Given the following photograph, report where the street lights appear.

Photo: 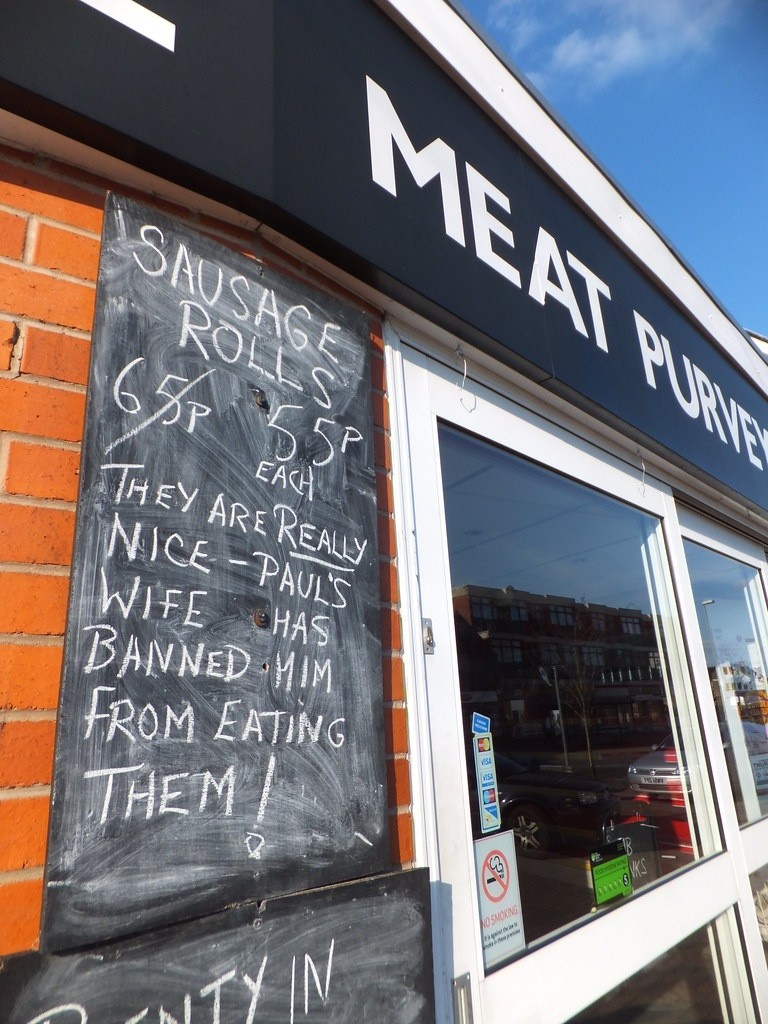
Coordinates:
[702,599,720,666]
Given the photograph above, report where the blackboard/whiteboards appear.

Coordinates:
[34,190,403,953]
[2,865,437,1024]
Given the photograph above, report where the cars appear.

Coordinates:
[465,744,621,860]
[625,720,768,800]
